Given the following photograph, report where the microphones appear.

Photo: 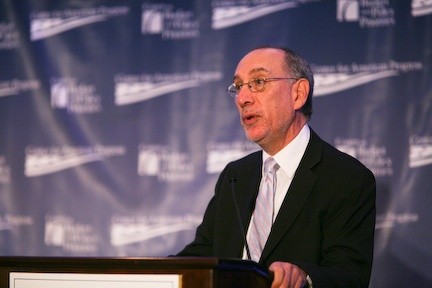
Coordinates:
[231,177,252,260]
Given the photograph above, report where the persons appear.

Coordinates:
[169,48,377,288]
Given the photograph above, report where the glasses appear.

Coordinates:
[225,77,298,98]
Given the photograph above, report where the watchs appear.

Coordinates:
[303,273,312,288]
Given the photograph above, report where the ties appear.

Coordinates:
[242,156,282,263]
